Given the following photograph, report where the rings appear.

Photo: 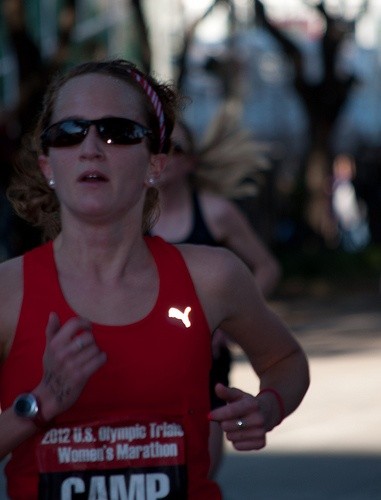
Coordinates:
[233,417,248,431]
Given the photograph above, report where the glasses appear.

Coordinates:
[38,118,155,148]
[167,142,188,156]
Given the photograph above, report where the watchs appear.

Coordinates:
[13,391,48,430]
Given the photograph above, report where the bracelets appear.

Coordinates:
[253,387,285,424]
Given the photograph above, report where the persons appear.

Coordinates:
[2,59,311,498]
[305,148,371,286]
[141,117,282,476]
